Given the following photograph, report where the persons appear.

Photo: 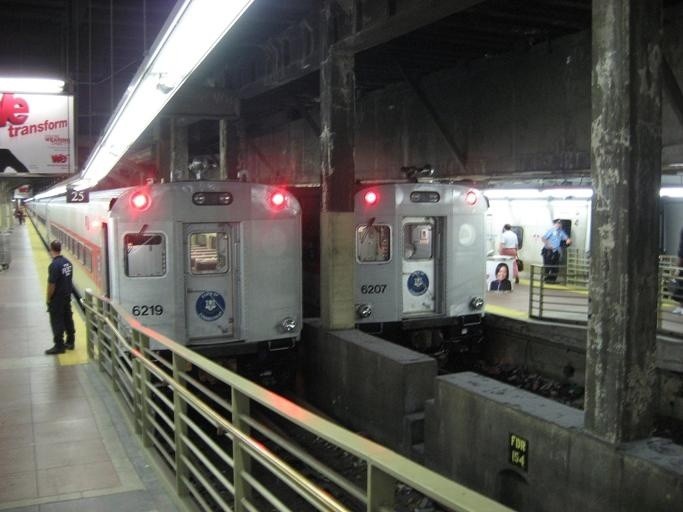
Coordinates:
[672,228,683,316]
[540,218,572,283]
[490,263,512,291]
[14,206,23,225]
[501,224,520,284]
[45,239,73,354]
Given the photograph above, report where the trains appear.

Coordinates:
[23,177,304,389]
[279,165,491,371]
[485,190,683,296]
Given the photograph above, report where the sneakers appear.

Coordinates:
[45,341,74,354]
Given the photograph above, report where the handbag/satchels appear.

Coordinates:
[515,259,523,271]
